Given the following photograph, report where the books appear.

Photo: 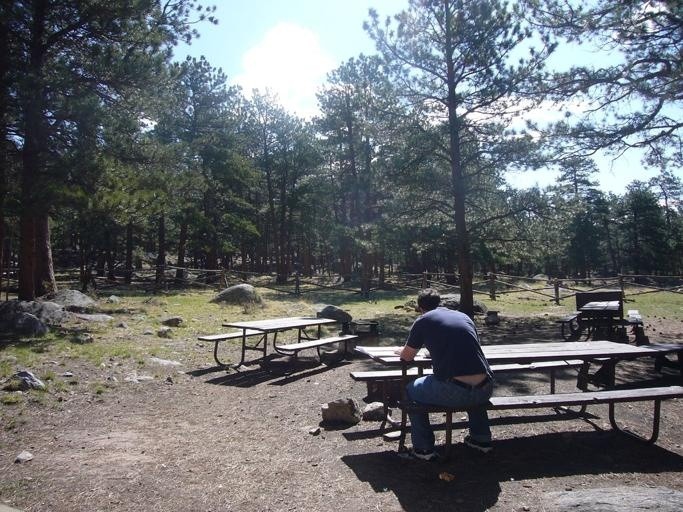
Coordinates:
[393,348,424,356]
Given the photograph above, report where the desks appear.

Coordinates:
[343,358,613,403]
[581,301,623,337]
[224,316,338,370]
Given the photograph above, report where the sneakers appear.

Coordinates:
[415,447,436,460]
[464,434,494,453]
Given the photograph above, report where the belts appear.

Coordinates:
[449,377,488,390]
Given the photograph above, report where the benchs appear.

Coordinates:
[396,383,681,451]
[272,332,362,373]
[198,329,270,367]
[556,309,582,335]
[625,307,644,338]
[351,338,654,434]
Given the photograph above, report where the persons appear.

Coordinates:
[399,287,495,463]
